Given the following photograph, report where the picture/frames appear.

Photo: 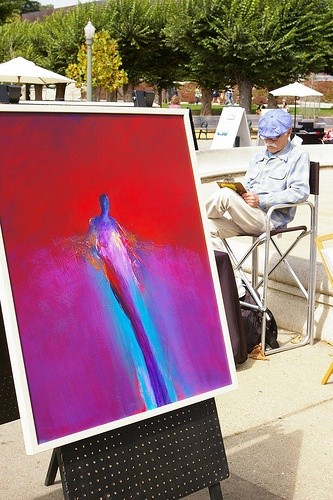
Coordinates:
[0,104,239,455]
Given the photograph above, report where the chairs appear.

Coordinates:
[219,161,320,356]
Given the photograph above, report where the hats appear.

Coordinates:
[258,109,293,138]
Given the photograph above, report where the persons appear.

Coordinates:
[282,99,288,109]
[257,101,265,110]
[171,92,180,105]
[194,86,200,104]
[323,129,333,140]
[212,87,234,105]
[205,108,310,301]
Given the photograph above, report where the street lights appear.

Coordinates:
[84,21,96,102]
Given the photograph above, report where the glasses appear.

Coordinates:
[259,134,281,141]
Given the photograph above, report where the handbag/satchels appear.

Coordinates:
[239,280,279,354]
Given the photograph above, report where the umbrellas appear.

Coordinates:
[269,82,323,128]
[0,56,77,87]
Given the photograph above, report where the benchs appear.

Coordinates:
[192,115,252,139]
